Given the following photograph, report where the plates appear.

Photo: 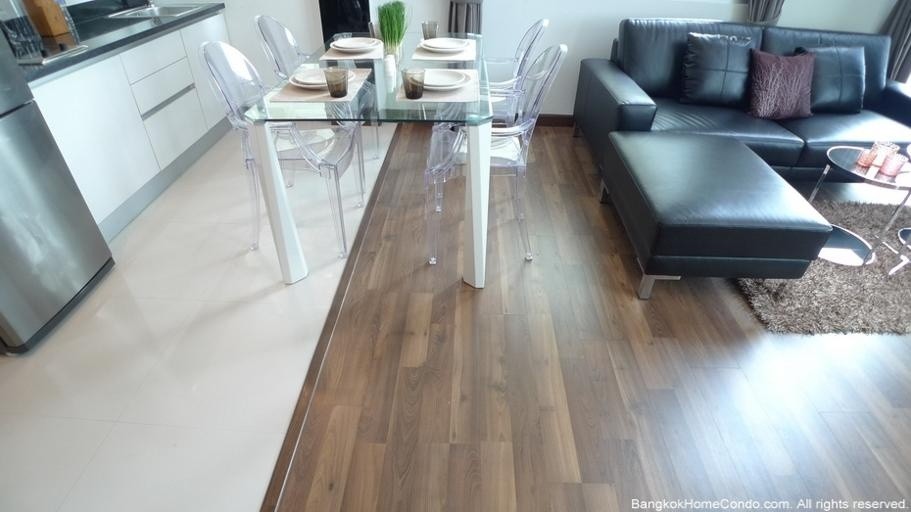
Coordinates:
[290,69,357,91]
[330,37,381,54]
[421,37,473,53]
[412,69,472,91]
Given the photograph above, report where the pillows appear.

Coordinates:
[748,44,816,123]
[799,45,868,116]
[675,27,757,106]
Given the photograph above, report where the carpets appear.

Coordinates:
[730,198,911,334]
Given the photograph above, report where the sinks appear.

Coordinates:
[104,4,206,18]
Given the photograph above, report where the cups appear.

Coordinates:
[422,20,439,40]
[402,66,426,99]
[325,66,348,98]
[367,20,386,41]
[859,141,909,176]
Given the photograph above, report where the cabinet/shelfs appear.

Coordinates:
[120,31,216,187]
[183,8,244,142]
[25,46,160,232]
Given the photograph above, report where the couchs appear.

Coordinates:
[570,15,911,301]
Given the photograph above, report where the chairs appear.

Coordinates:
[425,43,569,265]
[430,18,554,186]
[197,40,363,261]
[256,12,381,195]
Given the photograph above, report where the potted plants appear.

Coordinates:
[376,2,410,50]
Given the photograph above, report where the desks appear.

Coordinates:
[245,30,495,287]
[804,145,910,269]
